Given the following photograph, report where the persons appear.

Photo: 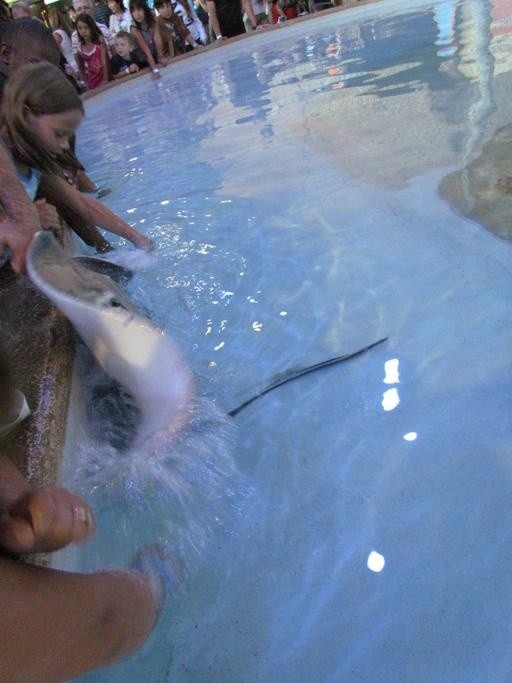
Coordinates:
[0,0,343,91]
[0,61,156,681]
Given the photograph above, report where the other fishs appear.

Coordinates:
[22,229,390,488]
